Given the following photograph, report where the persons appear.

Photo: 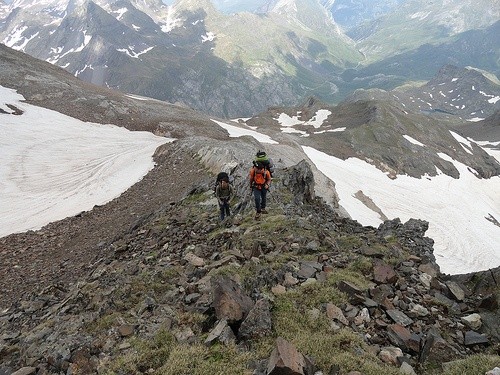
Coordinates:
[249,163,271,219]
[216,178,234,220]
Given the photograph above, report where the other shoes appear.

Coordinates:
[255,213,261,218]
[261,210,268,214]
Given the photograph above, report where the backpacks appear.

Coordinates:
[253,149,274,186]
[214,172,231,201]
